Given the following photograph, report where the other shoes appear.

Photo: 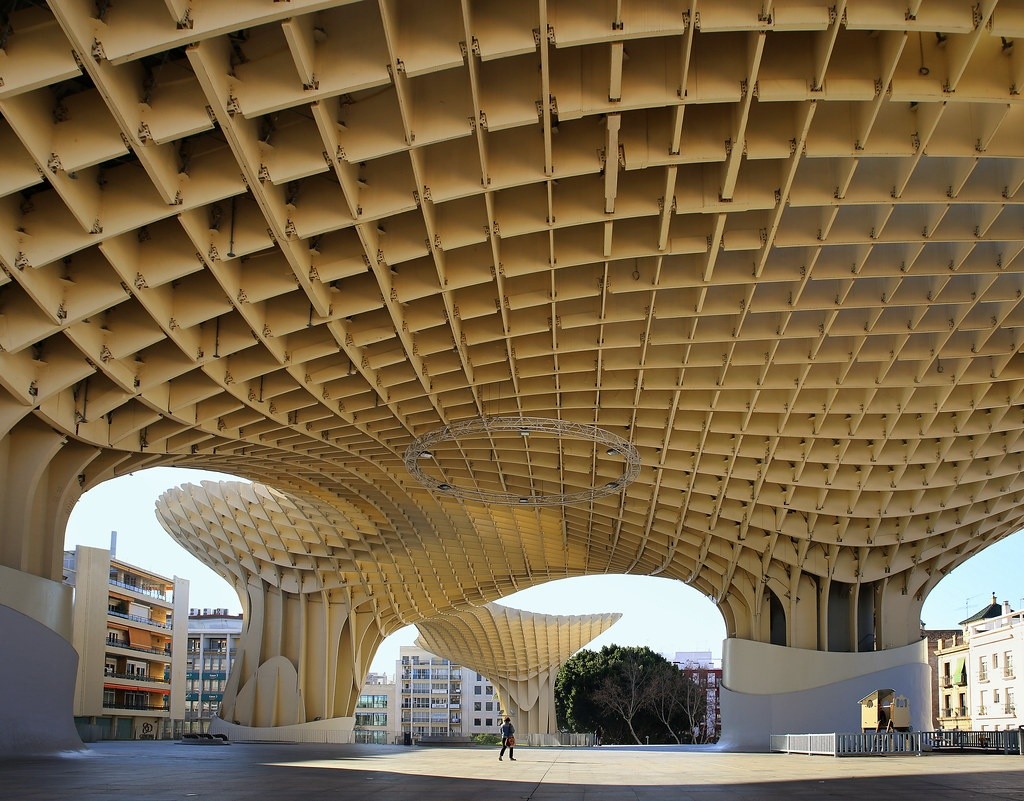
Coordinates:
[499,756,503,761]
[510,758,516,761]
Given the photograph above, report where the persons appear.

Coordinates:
[499,716,516,761]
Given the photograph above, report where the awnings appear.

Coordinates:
[953,658,964,682]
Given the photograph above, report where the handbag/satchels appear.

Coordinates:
[505,737,515,748]
